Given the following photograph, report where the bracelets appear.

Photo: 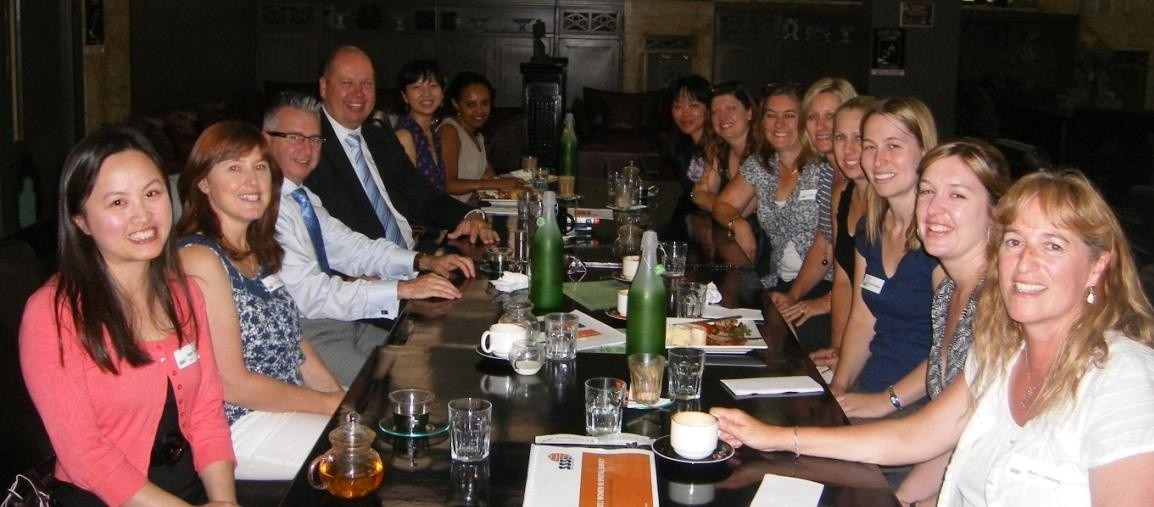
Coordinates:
[727,215,741,238]
[794,425,800,458]
[886,386,904,413]
[414,250,426,273]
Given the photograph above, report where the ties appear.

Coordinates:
[345,133,409,250]
[293,185,334,277]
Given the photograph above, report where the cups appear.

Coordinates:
[546,360,576,391]
[481,324,528,357]
[608,171,618,195]
[451,463,490,506]
[661,240,688,276]
[509,343,544,375]
[449,397,492,462]
[389,437,435,473]
[667,348,705,399]
[628,353,665,404]
[677,282,707,318]
[617,289,632,316]
[670,411,719,460]
[623,256,640,278]
[520,156,536,171]
[668,483,715,505]
[533,166,548,191]
[531,202,543,217]
[517,193,531,220]
[585,377,627,435]
[488,248,509,280]
[480,375,510,395]
[388,389,435,429]
[616,182,631,207]
[544,313,578,359]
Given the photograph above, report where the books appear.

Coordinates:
[563,209,614,224]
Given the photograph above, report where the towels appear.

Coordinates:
[488,269,531,294]
[566,208,614,220]
[702,280,724,303]
[720,375,826,396]
[510,169,532,181]
[749,471,825,507]
[701,303,764,322]
[479,206,519,215]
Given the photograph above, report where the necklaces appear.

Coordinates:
[239,250,261,274]
[1019,344,1050,406]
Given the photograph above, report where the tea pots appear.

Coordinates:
[620,160,644,195]
[308,412,384,497]
[616,217,644,259]
[498,301,540,346]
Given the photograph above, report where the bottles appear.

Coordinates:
[529,190,565,309]
[626,230,666,365]
[559,112,578,179]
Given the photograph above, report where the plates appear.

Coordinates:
[481,191,517,207]
[605,308,631,320]
[379,418,449,437]
[652,463,735,484]
[612,274,636,283]
[651,435,734,464]
[475,345,512,360]
[664,317,769,354]
[500,173,557,185]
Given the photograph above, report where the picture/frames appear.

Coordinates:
[870,26,906,76]
[899,0,935,28]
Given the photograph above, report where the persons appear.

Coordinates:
[303,44,502,252]
[709,74,832,304]
[805,97,883,368]
[833,139,1014,507]
[392,60,446,194]
[824,97,950,398]
[692,81,769,258]
[171,120,350,482]
[667,74,714,195]
[765,77,858,349]
[18,125,233,506]
[435,76,525,202]
[705,172,1154,507]
[262,89,475,388]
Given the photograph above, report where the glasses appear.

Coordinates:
[266,130,327,144]
